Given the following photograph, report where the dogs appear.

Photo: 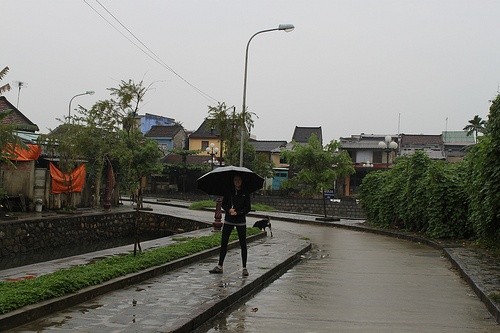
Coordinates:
[253,215,272,238]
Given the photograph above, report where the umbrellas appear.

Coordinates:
[196,165,264,193]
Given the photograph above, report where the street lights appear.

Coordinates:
[206,143,220,170]
[378,135,398,169]
[239,23,294,166]
[69,91,95,123]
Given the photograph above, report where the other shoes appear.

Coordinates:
[242,268,249,276]
[208,266,224,274]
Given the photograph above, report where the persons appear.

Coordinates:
[208,173,251,277]
